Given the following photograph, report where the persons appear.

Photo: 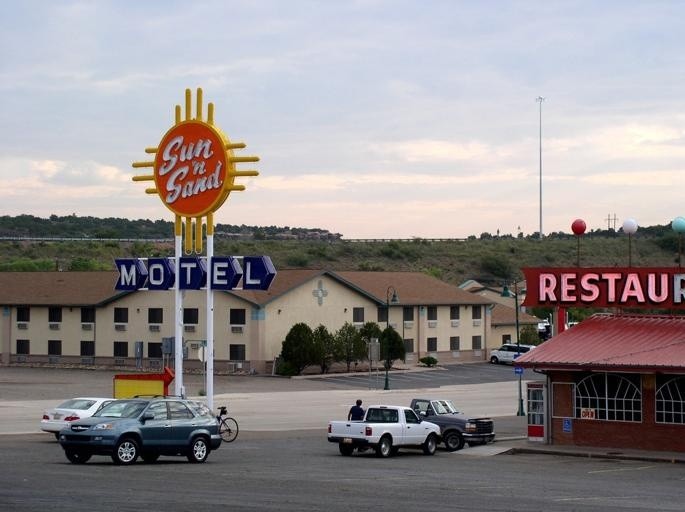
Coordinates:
[348,399,365,421]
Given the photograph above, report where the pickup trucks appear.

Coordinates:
[409,397,498,453]
[325,404,444,457]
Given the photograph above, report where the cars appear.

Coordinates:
[41,396,126,442]
[538,316,581,340]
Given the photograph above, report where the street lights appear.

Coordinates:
[384,284,401,392]
[499,275,530,416]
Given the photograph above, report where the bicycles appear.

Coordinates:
[213,406,238,443]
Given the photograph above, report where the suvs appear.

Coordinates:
[57,393,223,466]
[491,343,537,366]
